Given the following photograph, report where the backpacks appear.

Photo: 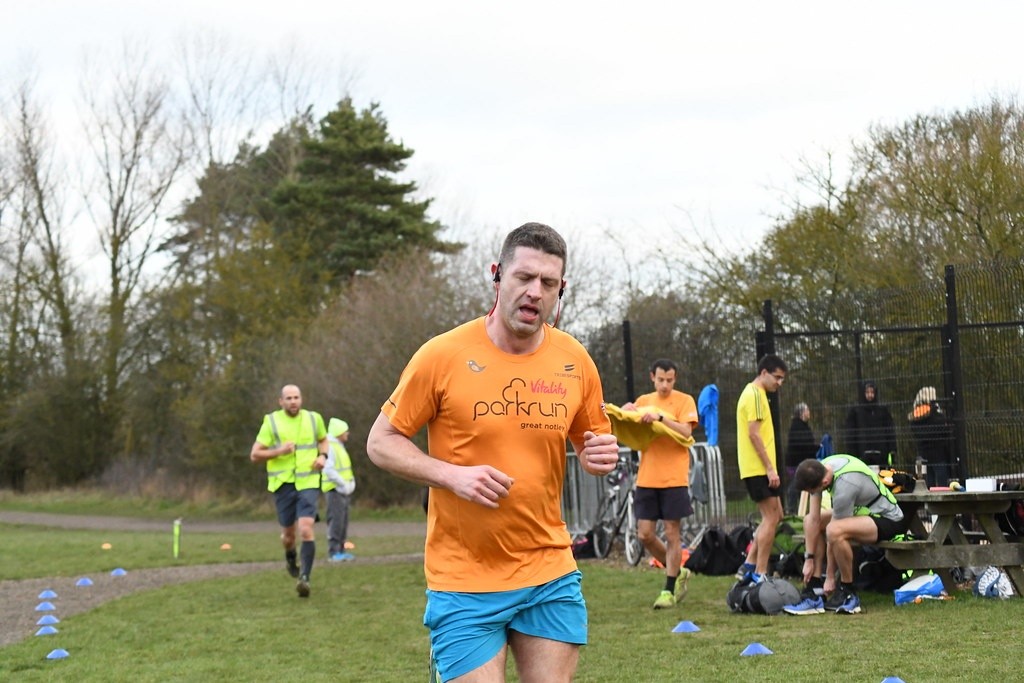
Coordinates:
[686,527,752,575]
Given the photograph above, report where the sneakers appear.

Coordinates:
[734,563,753,584]
[673,567,690,602]
[653,590,677,608]
[836,593,862,613]
[782,596,826,615]
[749,574,771,587]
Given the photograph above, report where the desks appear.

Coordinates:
[894,490,1024,598]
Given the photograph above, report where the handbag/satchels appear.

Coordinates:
[726,577,802,614]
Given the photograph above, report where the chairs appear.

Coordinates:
[733,484,804,579]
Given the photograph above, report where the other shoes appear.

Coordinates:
[328,551,354,563]
[296,581,309,597]
[287,563,299,577]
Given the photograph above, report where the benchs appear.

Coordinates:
[791,530,1024,551]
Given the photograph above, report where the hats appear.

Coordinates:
[328,417,348,438]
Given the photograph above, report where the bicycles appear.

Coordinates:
[592,457,643,566]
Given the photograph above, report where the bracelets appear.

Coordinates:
[804,552,814,559]
[318,453,328,459]
[658,412,663,421]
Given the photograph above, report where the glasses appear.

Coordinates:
[770,371,784,382]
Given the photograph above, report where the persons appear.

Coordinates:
[322,417,355,561]
[845,381,897,465]
[251,384,329,597]
[912,387,949,491]
[619,359,698,607]
[787,404,820,515]
[783,454,907,614]
[369,222,619,683]
[735,355,785,581]
